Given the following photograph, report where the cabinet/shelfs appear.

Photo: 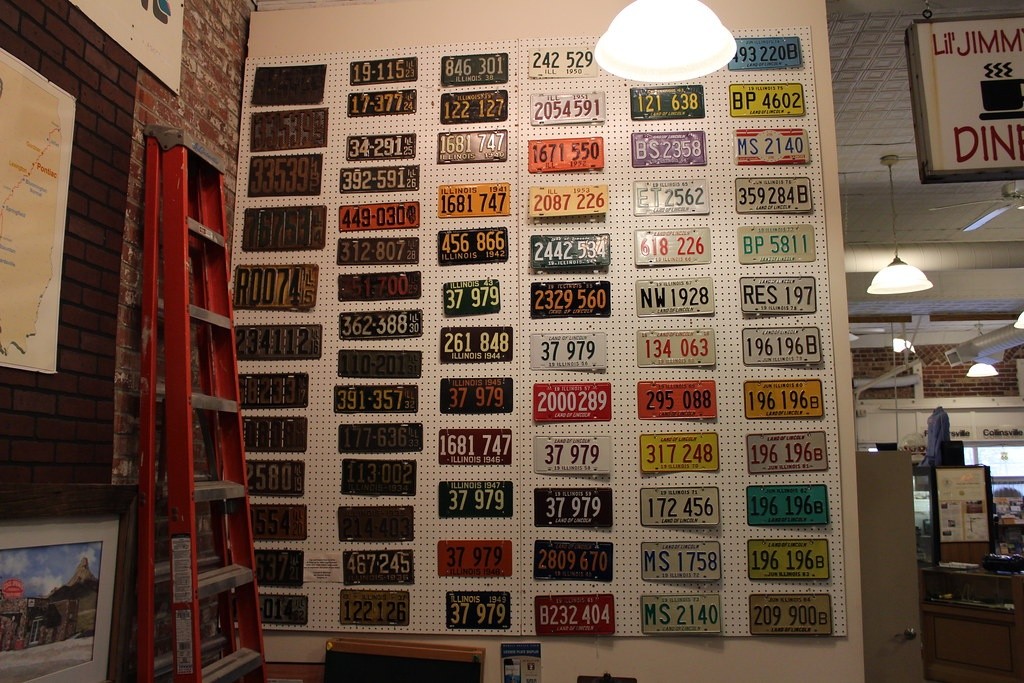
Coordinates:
[918,563,1024,683]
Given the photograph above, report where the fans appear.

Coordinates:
[928,182,1024,232]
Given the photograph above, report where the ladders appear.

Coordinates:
[135,124,269,683]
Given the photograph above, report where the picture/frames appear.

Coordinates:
[0,481,164,683]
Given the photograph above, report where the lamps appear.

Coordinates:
[594,0,738,83]
[965,320,999,377]
[866,155,935,295]
